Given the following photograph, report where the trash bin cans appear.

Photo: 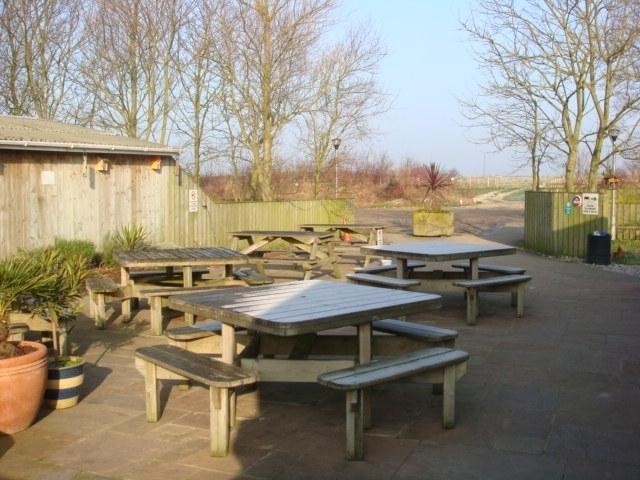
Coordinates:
[587,233,611,265]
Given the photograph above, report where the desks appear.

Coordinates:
[298,224,386,246]
[168,278,444,458]
[113,246,249,325]
[361,239,516,324]
[230,230,341,280]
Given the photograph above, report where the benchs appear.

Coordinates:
[130,269,210,283]
[451,262,526,307]
[134,345,256,455]
[375,319,457,350]
[345,272,421,290]
[164,322,246,350]
[316,346,468,460]
[139,283,241,335]
[85,276,118,331]
[354,263,426,279]
[453,273,532,326]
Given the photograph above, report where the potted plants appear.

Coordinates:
[412,162,455,236]
[17,238,95,410]
[0,255,65,435]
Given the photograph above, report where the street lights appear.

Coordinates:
[332,139,341,197]
[608,129,620,240]
